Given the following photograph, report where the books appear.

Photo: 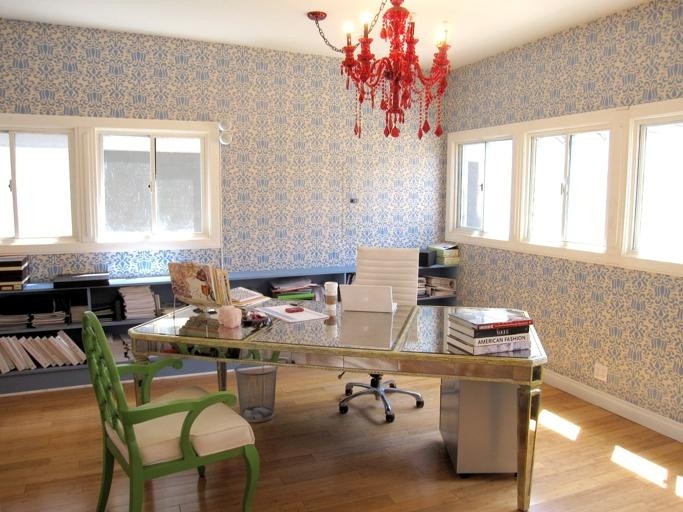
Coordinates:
[92,305,113,322]
[269,277,324,301]
[70,305,89,322]
[447,307,531,357]
[418,244,460,300]
[31,311,70,329]
[168,262,272,307]
[119,285,188,320]
[2,314,30,331]
[105,333,137,363]
[2,330,87,374]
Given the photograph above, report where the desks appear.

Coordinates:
[128,299,548,509]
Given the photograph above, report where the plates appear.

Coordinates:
[241,313,268,326]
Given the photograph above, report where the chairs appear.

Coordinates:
[338,246,424,422]
[81,311,260,512]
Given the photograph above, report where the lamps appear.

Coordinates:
[341,0,453,138]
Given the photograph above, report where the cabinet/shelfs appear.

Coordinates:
[0,264,461,396]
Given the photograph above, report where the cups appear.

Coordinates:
[323,281,338,310]
[217,305,241,329]
[322,311,337,339]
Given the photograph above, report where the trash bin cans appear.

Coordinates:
[235,364,278,423]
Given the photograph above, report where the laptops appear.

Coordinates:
[340,305,398,351]
[339,283,398,312]
